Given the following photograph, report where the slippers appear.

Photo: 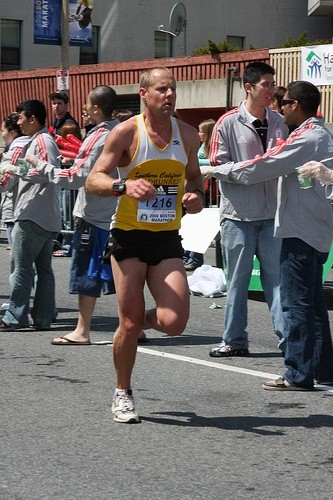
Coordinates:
[51,332,91,345]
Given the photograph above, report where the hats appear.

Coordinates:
[48,91,69,102]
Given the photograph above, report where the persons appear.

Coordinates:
[25,85,149,345]
[84,67,204,423]
[268,86,299,135]
[0,99,61,332]
[199,81,333,391]
[209,62,289,358]
[295,160,333,185]
[48,91,135,168]
[52,123,82,257]
[181,119,215,271]
[0,113,59,323]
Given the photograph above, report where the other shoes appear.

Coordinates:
[262,376,310,391]
[53,250,70,256]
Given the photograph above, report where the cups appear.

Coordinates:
[295,166,314,189]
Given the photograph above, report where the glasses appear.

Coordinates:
[252,81,276,90]
[280,99,294,106]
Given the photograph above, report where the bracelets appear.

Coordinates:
[193,189,204,199]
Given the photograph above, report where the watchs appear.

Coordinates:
[112,178,129,194]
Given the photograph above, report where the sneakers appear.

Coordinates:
[182,255,204,270]
[210,341,249,356]
[111,388,141,423]
[0,321,17,331]
